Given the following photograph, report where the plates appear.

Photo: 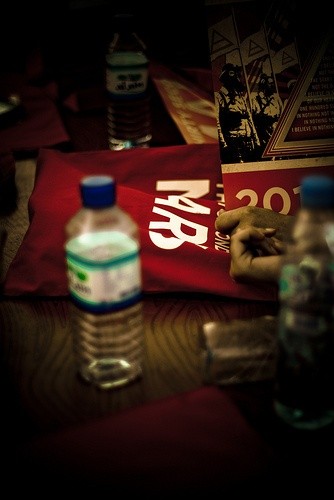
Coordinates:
[0,97,22,122]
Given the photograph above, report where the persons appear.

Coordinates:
[214,62,298,164]
[215,205,297,287]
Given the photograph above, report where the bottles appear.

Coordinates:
[105,12,153,151]
[64,175,145,390]
[273,176,334,430]
